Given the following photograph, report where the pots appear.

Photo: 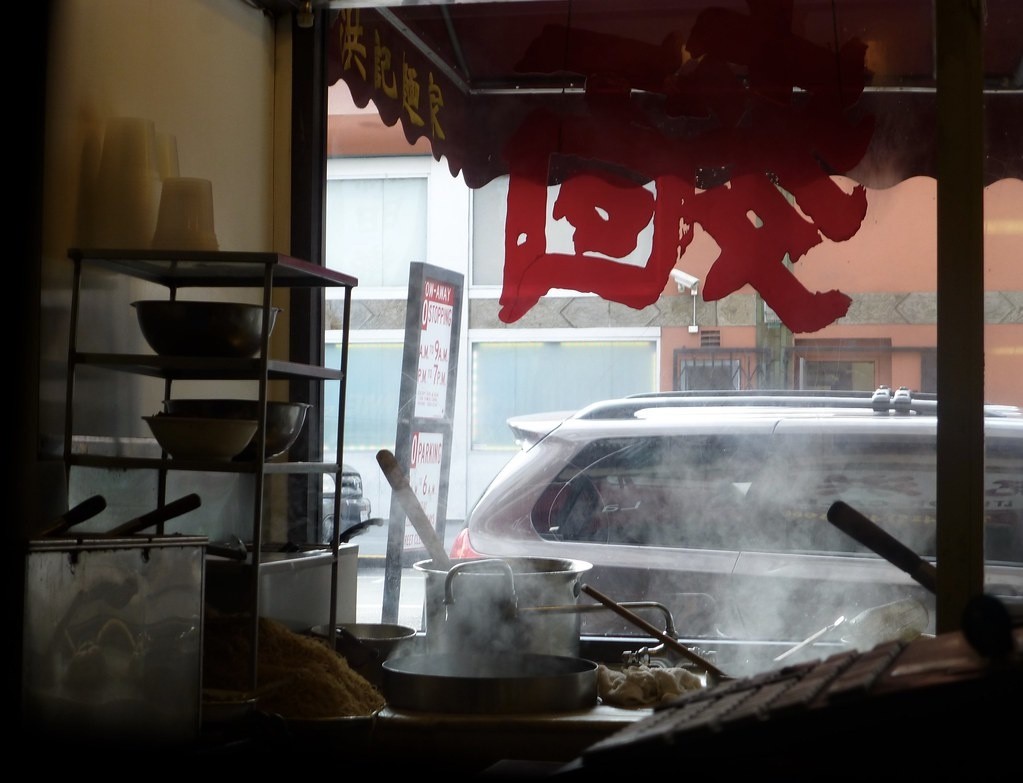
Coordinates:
[312,621,416,682]
[380,653,598,716]
[417,555,594,654]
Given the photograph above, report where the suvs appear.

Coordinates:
[446,390,1023,671]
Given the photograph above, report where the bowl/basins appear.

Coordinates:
[161,399,314,461]
[145,412,259,465]
[129,299,283,359]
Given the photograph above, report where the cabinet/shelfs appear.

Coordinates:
[59,252,358,738]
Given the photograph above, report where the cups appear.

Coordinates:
[88,114,219,252]
